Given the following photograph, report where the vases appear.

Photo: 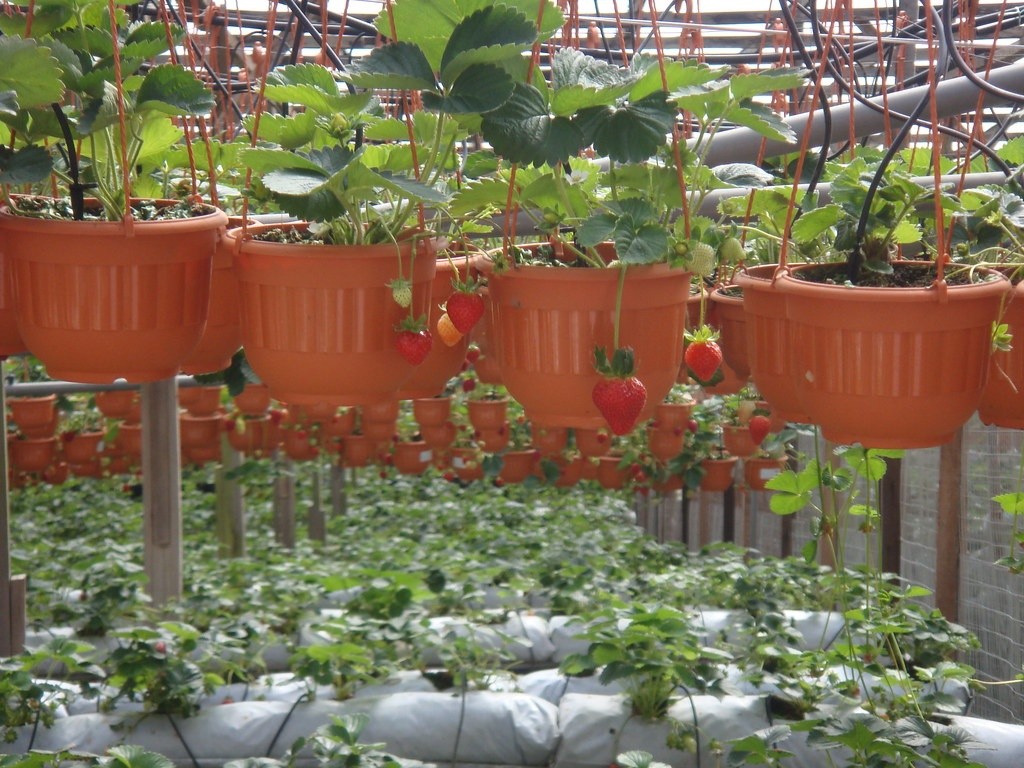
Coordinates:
[179,386,225,416]
[285,402,338,420]
[5,418,791,492]
[363,400,400,424]
[178,217,261,375]
[234,387,271,416]
[95,390,136,418]
[732,261,809,401]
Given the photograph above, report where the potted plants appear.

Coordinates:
[655,383,696,434]
[718,389,770,458]
[708,184,836,385]
[220,0,568,384]
[0,355,58,437]
[0,0,229,386]
[775,155,1013,447]
[462,384,509,431]
[412,377,455,427]
[482,49,789,431]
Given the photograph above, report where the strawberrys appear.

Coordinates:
[219,277,771,497]
